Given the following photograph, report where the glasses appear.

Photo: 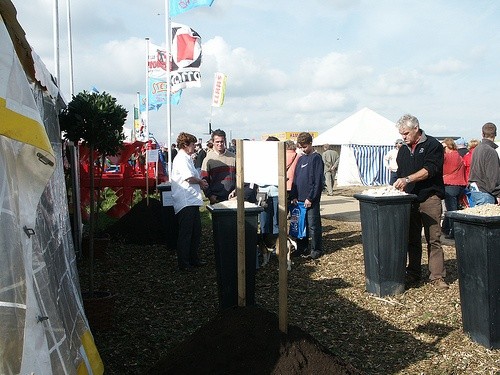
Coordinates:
[443,146,447,150]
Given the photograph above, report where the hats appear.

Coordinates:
[396,138,404,143]
[456,138,467,146]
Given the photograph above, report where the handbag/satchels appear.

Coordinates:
[288,201,308,238]
[256,192,269,208]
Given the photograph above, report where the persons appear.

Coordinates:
[201,129,237,204]
[384,138,404,185]
[281,140,299,217]
[159,139,236,173]
[468,123,500,207]
[168,133,208,275]
[258,137,285,257]
[439,135,481,240]
[391,115,449,291]
[289,133,323,262]
[242,138,258,204]
[322,144,339,196]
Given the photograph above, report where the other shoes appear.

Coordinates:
[405,272,424,283]
[293,243,308,256]
[327,194,334,196]
[430,277,449,289]
[180,264,192,272]
[445,233,454,239]
[300,248,324,259]
[191,260,207,266]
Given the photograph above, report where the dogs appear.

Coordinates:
[256,233,297,272]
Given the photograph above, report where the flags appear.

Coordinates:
[170,21,203,95]
[148,77,182,105]
[138,95,161,113]
[148,43,171,81]
[130,108,146,144]
[209,72,225,107]
[168,0,214,17]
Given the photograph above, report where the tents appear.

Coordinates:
[316,107,408,188]
